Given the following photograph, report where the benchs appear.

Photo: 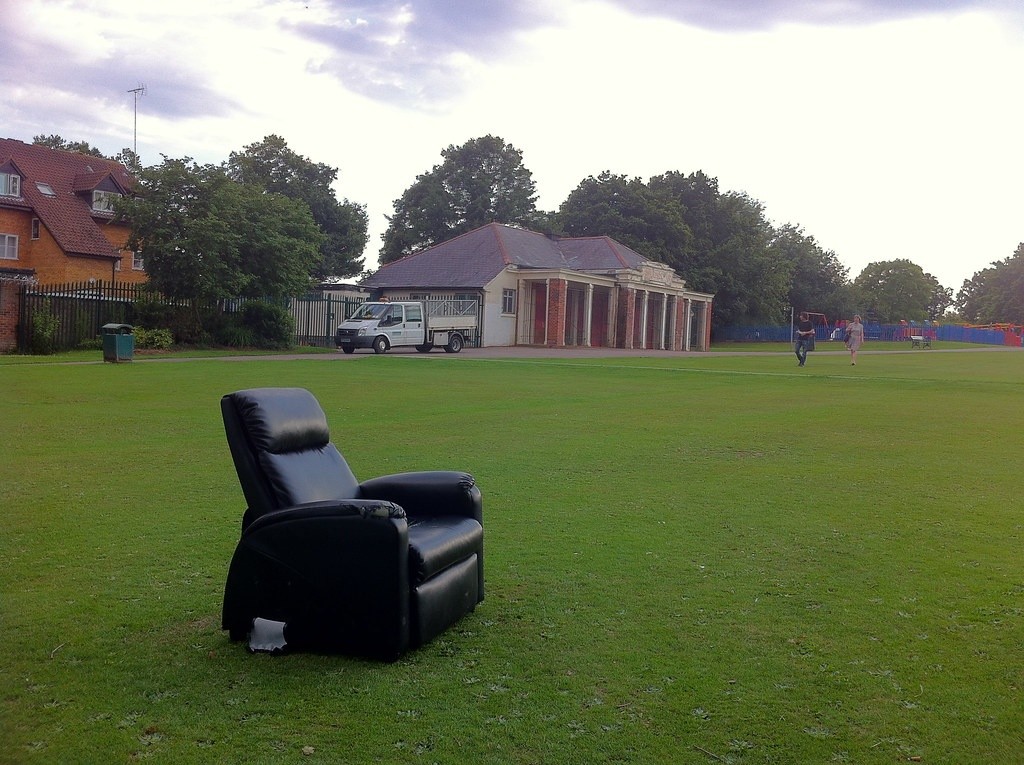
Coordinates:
[911,336,932,349]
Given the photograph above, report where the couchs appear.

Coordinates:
[217,388,485,665]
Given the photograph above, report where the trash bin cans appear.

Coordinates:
[102,323,135,363]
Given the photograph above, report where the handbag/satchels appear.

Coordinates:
[843,333,850,343]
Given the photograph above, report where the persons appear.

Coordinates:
[846,315,864,366]
[794,312,815,367]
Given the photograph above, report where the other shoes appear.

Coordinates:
[851,362,855,365]
[798,362,802,365]
[801,363,805,367]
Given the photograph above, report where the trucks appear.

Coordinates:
[330,295,477,354]
